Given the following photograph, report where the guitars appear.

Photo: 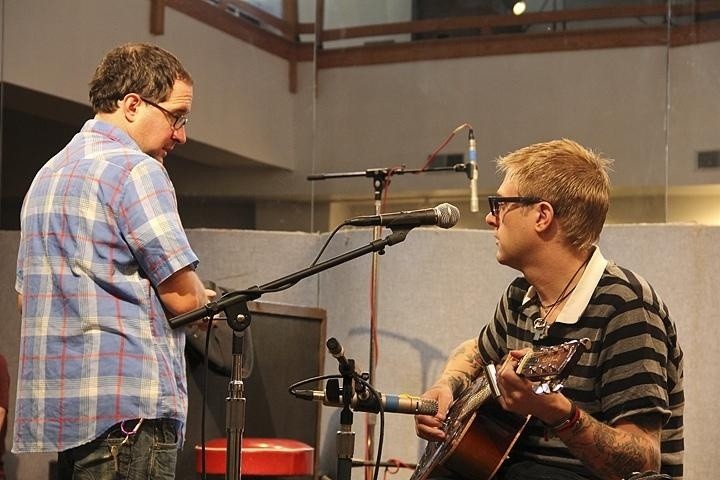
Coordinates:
[411,336,592,480]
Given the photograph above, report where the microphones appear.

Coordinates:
[294,387,439,416]
[344,201,461,230]
[467,128,479,213]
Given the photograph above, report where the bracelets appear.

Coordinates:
[546,398,576,431]
[552,406,581,434]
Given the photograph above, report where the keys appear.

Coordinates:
[110,444,122,473]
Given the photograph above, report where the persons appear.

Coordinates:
[409,134,685,479]
[7,42,215,480]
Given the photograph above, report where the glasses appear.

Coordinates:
[118,90,190,132]
[486,190,558,218]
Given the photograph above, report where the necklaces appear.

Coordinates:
[538,286,576,308]
[533,255,592,341]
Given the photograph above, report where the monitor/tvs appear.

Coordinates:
[178,305,329,477]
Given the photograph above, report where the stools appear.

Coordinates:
[193,435,316,480]
